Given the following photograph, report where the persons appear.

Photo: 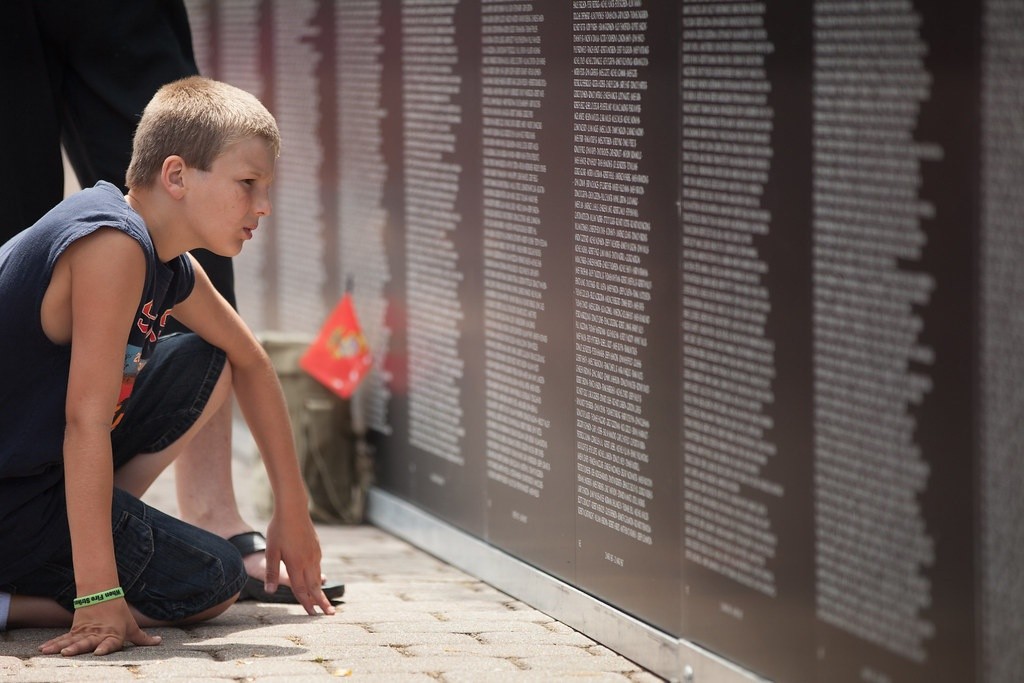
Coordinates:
[0,1,346,601]
[0,77,335,655]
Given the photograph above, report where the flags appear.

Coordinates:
[302,292,375,402]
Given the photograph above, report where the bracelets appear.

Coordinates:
[74,587,125,608]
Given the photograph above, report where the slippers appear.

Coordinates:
[229,532,345,604]
